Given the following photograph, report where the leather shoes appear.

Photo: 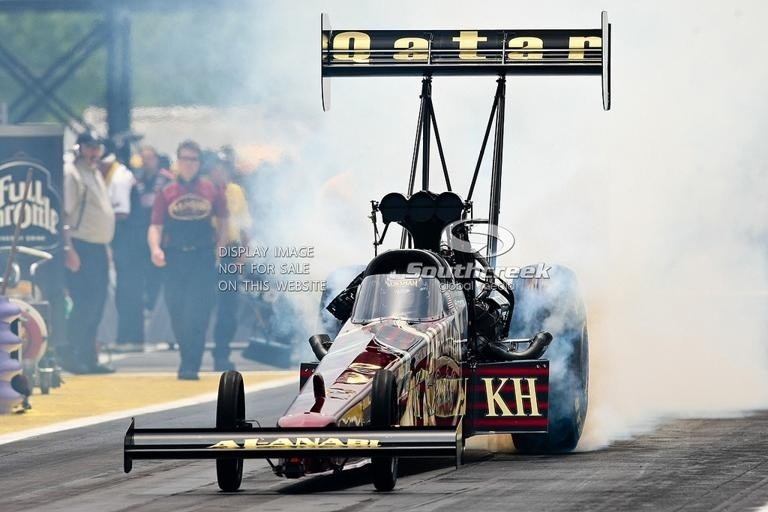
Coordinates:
[63,343,238,381]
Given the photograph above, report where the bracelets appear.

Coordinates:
[62,243,77,252]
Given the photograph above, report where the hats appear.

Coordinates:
[76,130,105,147]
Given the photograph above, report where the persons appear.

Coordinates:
[200,150,256,373]
[367,265,428,318]
[60,128,116,377]
[144,138,231,381]
[101,130,306,351]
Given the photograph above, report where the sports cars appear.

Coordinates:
[120,9,617,496]
